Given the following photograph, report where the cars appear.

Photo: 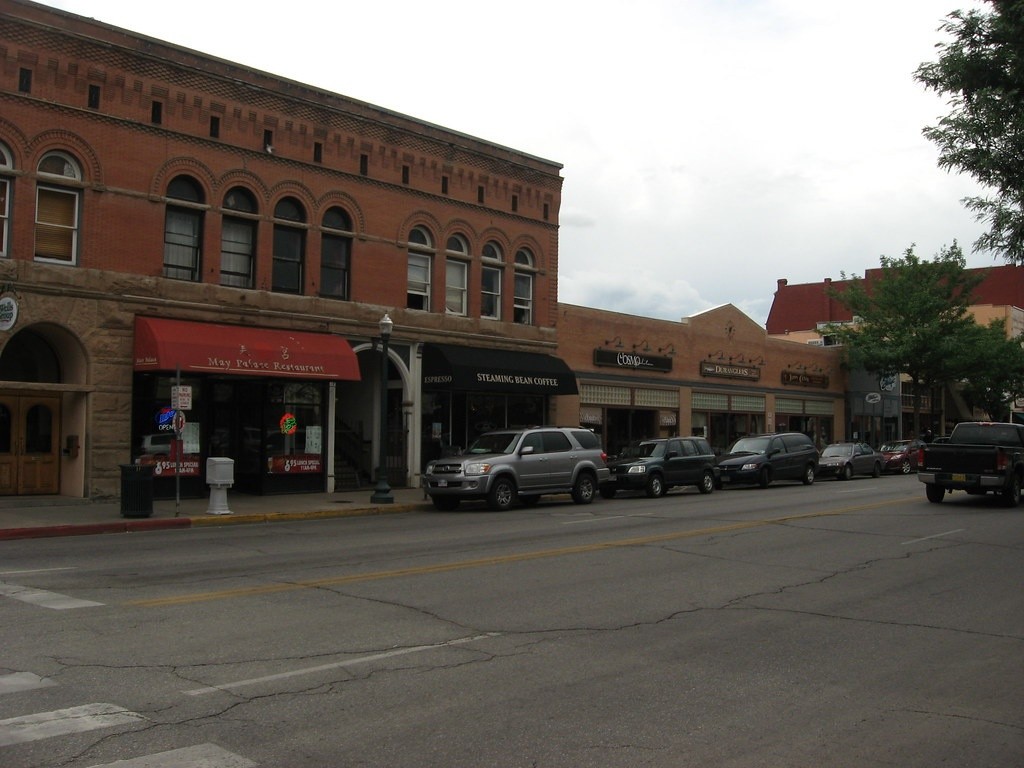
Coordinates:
[819,441,886,480]
[878,437,950,474]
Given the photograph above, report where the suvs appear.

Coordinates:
[422,425,609,511]
[600,436,721,499]
[714,432,820,490]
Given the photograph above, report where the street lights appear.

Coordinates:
[371,309,394,504]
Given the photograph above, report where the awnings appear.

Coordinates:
[133,316,361,380]
[421,342,578,395]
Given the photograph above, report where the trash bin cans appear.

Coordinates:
[119,462,158,518]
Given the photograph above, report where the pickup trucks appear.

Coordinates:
[918,421,1024,507]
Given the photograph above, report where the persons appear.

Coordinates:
[923,430,934,443]
